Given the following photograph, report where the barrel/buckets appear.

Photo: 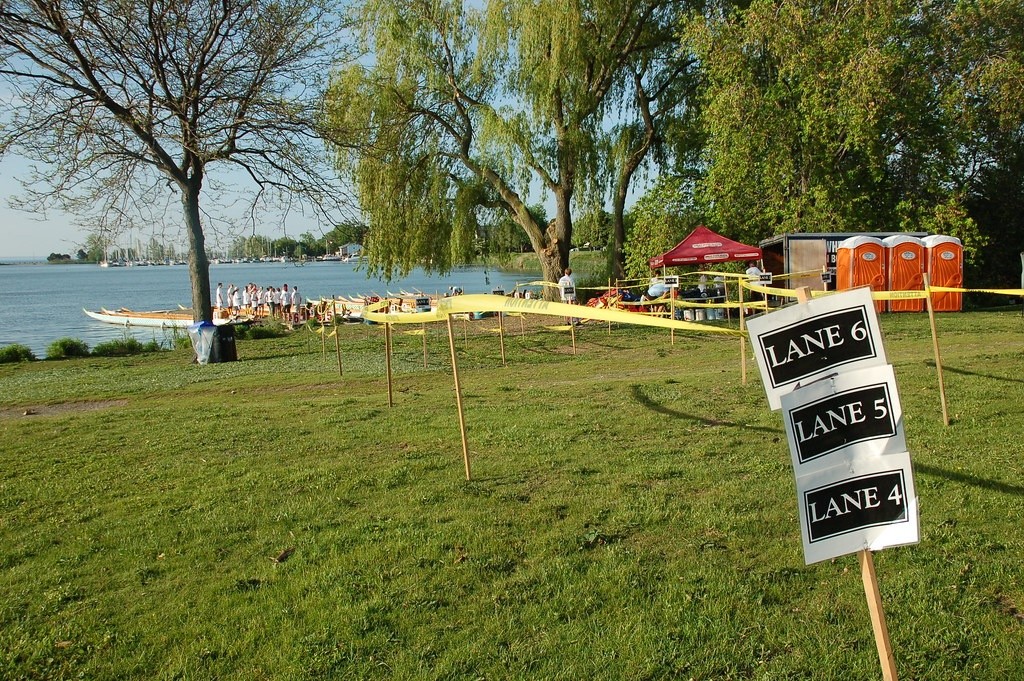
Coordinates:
[695,309,706,321]
[715,309,725,320]
[473,310,482,319]
[707,308,716,321]
[683,309,694,321]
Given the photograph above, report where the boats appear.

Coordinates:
[80,286,470,326]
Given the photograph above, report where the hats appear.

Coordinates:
[234,287,238,290]
[449,287,452,290]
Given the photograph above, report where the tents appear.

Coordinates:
[647,223,764,322]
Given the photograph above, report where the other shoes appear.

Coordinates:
[568,322,571,325]
[576,322,583,326]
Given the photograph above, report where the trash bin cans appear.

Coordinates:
[187,320,237,365]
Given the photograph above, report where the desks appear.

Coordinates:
[682,295,726,320]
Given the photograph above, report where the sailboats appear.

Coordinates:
[99,232,189,267]
[207,236,369,264]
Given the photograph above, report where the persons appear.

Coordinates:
[243,282,302,320]
[647,282,670,317]
[746,259,765,282]
[697,268,710,292]
[444,292,451,298]
[649,270,661,312]
[233,287,241,315]
[558,268,576,303]
[712,269,724,288]
[217,283,223,311]
[449,286,462,296]
[227,283,234,315]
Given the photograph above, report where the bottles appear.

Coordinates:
[717,288,720,296]
